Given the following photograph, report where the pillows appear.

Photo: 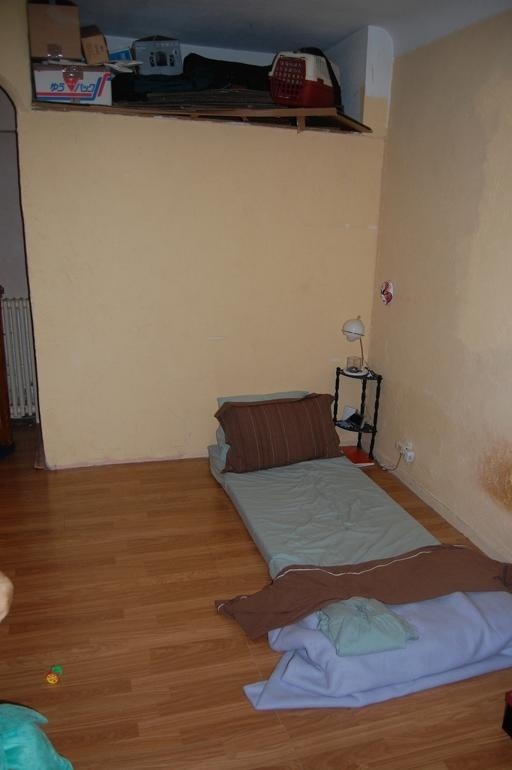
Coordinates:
[215,392,343,473]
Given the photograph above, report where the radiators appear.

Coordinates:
[0,297,39,420]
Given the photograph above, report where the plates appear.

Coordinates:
[343,366,369,377]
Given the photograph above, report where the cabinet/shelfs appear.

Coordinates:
[332,365,382,460]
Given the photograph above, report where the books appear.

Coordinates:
[340,444,377,467]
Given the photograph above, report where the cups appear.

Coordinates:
[347,355,363,371]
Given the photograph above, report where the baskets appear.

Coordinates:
[269,51,343,107]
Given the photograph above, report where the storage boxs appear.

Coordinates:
[28,4,184,107]
[268,43,341,107]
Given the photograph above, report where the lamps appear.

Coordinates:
[341,313,369,376]
